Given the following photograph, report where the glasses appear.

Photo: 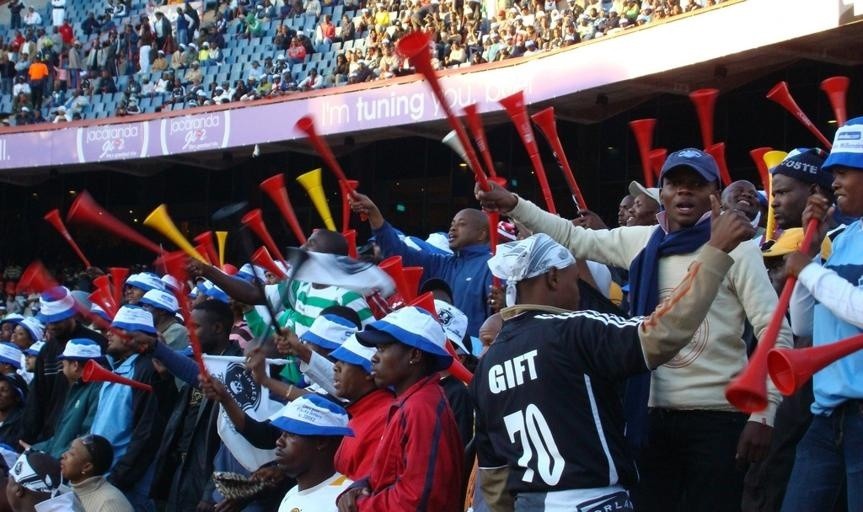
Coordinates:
[81,434,97,466]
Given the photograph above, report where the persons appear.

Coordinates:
[1,1,727,132]
[1,112,863,512]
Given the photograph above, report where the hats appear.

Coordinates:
[820,117,863,176]
[434,299,470,355]
[270,394,353,436]
[1,372,27,400]
[659,148,721,191]
[161,274,180,290]
[17,317,45,341]
[112,305,156,333]
[327,332,378,374]
[628,180,661,205]
[231,264,266,283]
[34,286,77,323]
[197,280,229,304]
[58,338,104,361]
[139,289,178,313]
[188,286,198,298]
[23,341,46,356]
[302,315,358,349]
[89,301,113,323]
[0,312,24,323]
[126,272,165,291]
[365,306,453,371]
[0,342,22,368]
[356,329,396,347]
[769,147,810,174]
